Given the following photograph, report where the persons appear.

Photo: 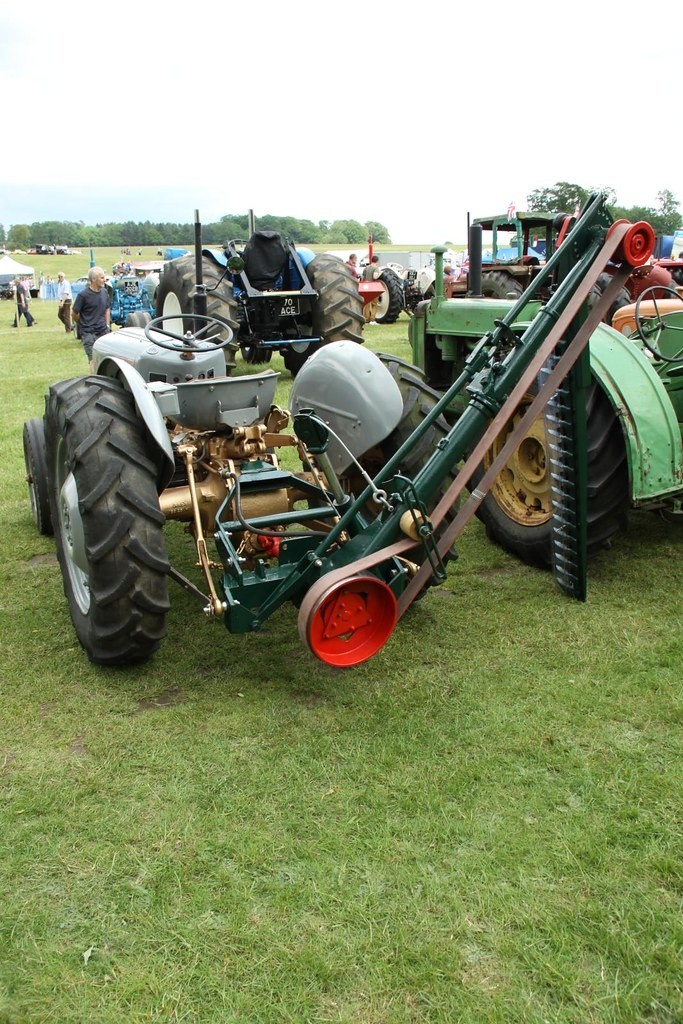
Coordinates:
[71,267,111,363]
[362,256,380,325]
[158,247,162,256]
[38,271,44,288]
[13,278,36,328]
[139,248,142,255]
[121,248,131,261]
[346,254,358,278]
[56,273,73,332]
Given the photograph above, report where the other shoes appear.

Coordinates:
[369,321,379,325]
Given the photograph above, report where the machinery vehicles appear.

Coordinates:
[22,192,683,668]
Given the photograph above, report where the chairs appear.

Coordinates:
[175,369,283,430]
[240,229,287,281]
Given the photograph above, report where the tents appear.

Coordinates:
[0,256,36,291]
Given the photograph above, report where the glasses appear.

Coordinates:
[58,276,63,278]
